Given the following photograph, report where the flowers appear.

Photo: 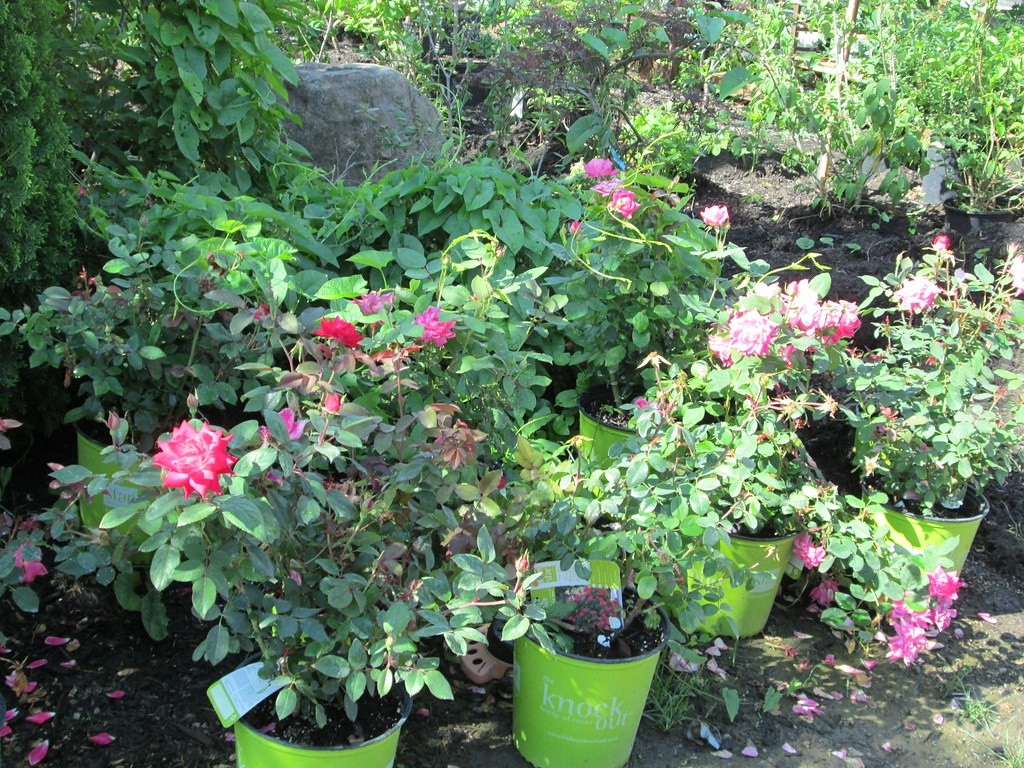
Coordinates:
[0,146,1024,666]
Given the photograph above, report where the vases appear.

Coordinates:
[860,471,991,580]
[232,649,412,768]
[511,584,670,768]
[577,385,638,468]
[674,524,799,640]
[78,431,153,534]
